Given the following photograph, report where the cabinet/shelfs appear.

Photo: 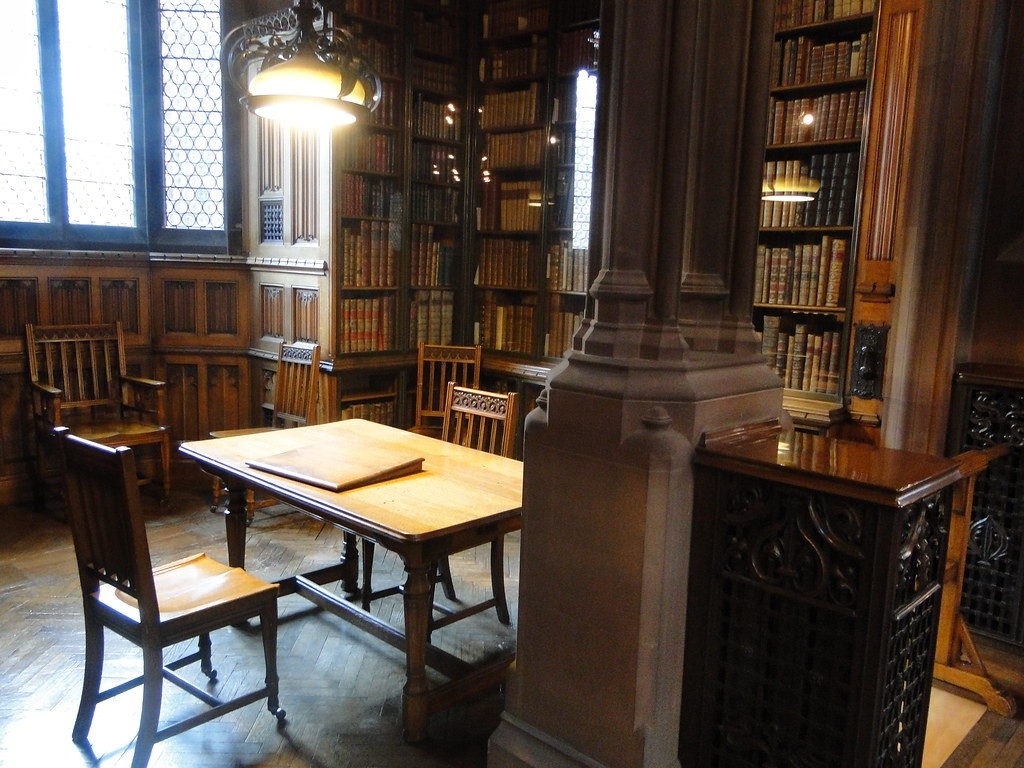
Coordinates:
[943,363,1023,653]
[240,0,470,429]
[678,418,964,768]
[459,1,667,462]
[753,0,883,432]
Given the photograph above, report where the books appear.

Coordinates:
[766,90,869,148]
[331,25,594,430]
[750,315,841,393]
[771,0,878,31]
[768,32,876,89]
[759,153,859,229]
[754,236,848,309]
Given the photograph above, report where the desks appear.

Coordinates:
[181,417,523,741]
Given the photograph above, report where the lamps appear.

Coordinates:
[219,1,383,129]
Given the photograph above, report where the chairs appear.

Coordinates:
[408,341,482,449]
[26,320,174,518]
[210,342,321,526]
[54,426,287,768]
[359,382,518,643]
